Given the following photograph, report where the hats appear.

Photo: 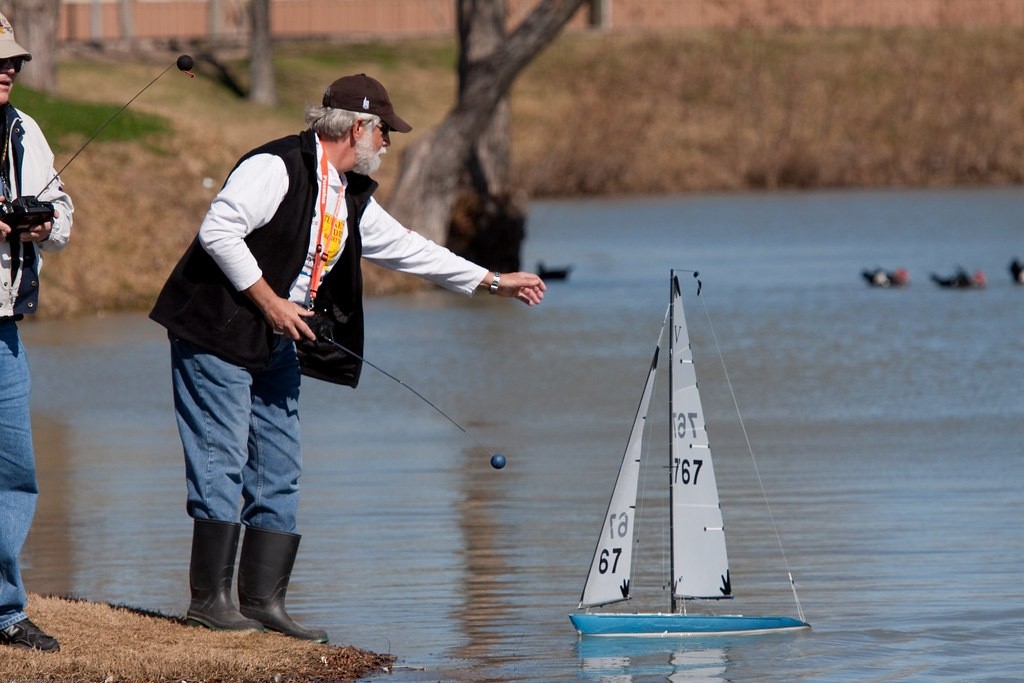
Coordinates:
[322,73,413,132]
[0,13,32,62]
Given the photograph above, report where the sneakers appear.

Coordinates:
[0,618,60,655]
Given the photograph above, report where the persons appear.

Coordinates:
[147,74,548,643]
[0,11,73,655]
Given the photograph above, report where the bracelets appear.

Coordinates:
[489,272,500,294]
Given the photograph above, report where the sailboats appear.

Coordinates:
[565,267,811,638]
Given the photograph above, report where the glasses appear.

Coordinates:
[0,58,22,72]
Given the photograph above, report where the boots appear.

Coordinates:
[239,527,330,643]
[187,519,264,633]
